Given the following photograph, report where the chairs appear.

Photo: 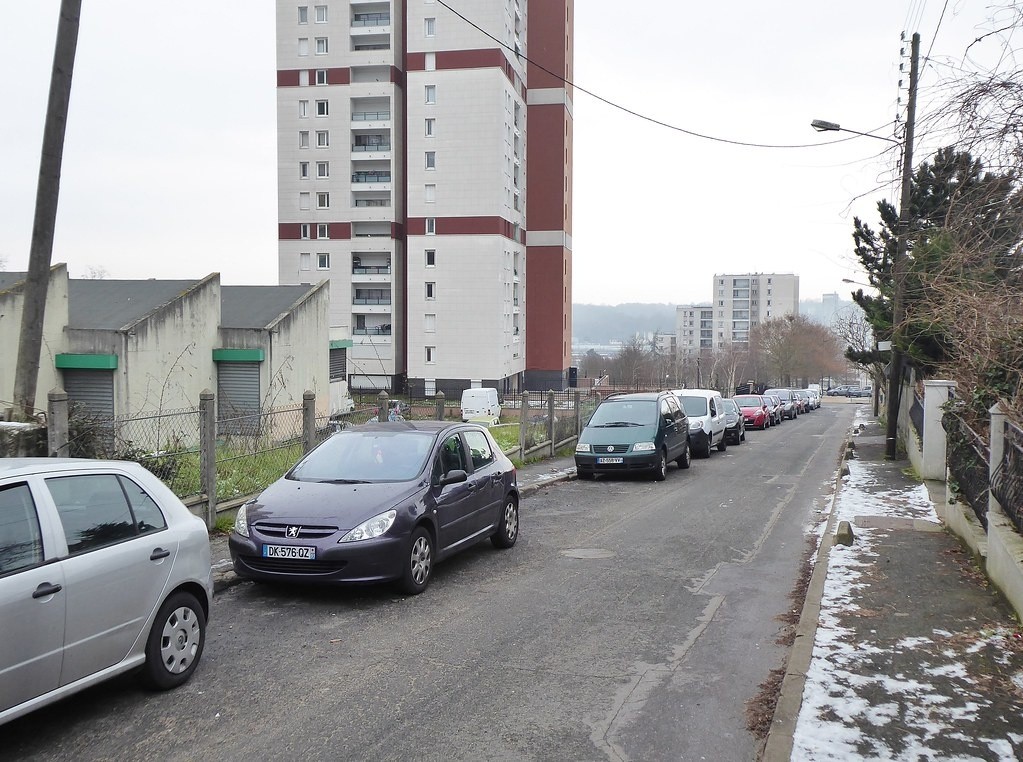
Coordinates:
[83,489,134,525]
[764,399,770,405]
[442,444,459,473]
[389,437,419,457]
[339,438,379,467]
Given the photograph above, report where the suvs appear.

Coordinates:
[229,420,519,596]
[0,455,216,730]
[574,390,693,481]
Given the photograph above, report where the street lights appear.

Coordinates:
[809,118,913,461]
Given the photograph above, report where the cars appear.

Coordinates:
[464,415,501,429]
[826,385,872,398]
[364,399,411,423]
[721,387,823,447]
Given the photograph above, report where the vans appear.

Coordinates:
[668,388,726,460]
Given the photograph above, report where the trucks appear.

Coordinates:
[460,387,504,421]
